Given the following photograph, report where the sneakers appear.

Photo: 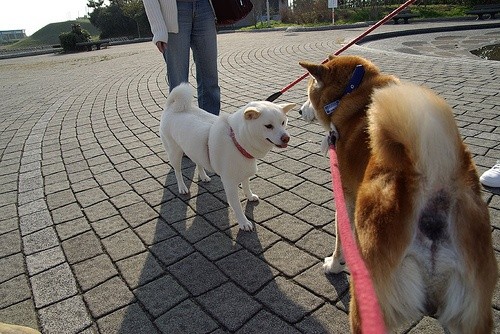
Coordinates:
[480,159,500,188]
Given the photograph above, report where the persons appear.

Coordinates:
[479,160,500,187]
[143,0,254,156]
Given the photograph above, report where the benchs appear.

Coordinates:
[75,40,110,50]
[392,13,420,24]
[464,4,500,21]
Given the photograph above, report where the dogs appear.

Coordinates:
[299,54,500,334]
[159,82,297,233]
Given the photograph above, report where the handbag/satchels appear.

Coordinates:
[210,0,253,24]
